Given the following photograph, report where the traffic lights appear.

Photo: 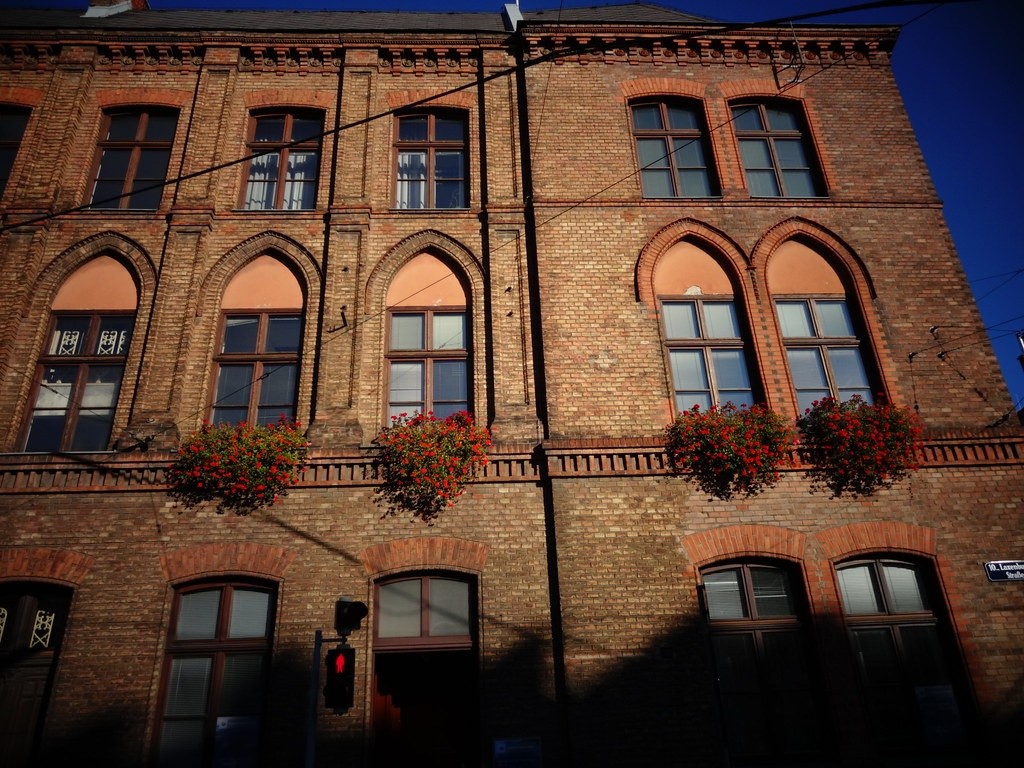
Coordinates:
[322,600,369,716]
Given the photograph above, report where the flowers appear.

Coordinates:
[380,409,495,508]
[165,414,311,505]
[796,392,934,482]
[663,402,800,496]
